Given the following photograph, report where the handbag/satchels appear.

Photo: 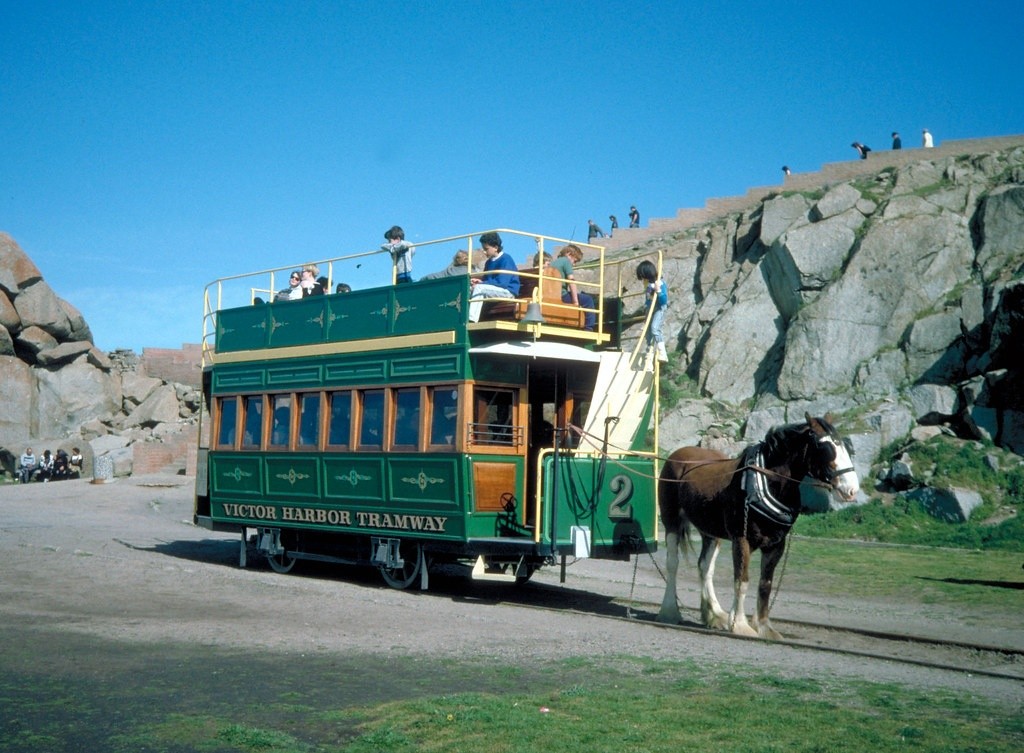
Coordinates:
[860,145,871,154]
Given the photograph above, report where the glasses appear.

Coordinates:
[291,276,300,281]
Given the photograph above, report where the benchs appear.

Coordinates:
[490,266,584,327]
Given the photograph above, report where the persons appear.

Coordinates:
[421,205,641,332]
[892,133,901,150]
[636,260,668,363]
[381,226,415,285]
[852,144,872,159]
[274,264,351,302]
[228,400,514,446]
[782,166,791,175]
[19,448,83,482]
[922,129,933,148]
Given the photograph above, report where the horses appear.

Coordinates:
[657,411,859,641]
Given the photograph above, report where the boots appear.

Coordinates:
[646,346,654,352]
[657,342,668,363]
